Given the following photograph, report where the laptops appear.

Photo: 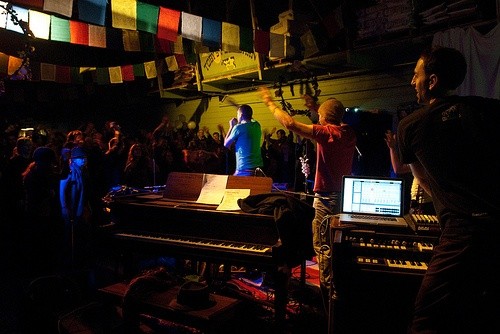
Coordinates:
[339,175,408,228]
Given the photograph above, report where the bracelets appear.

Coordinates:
[273,106,279,115]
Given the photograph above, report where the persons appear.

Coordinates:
[0,86,434,334]
[396,46,500,334]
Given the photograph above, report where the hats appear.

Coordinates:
[317,98,345,123]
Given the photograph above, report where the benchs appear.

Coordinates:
[97,277,241,334]
